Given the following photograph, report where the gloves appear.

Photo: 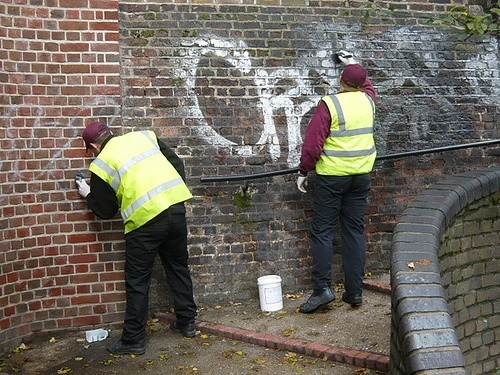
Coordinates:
[75,179,91,198]
[297,176,308,194]
[337,50,356,66]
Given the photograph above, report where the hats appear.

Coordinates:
[82,122,110,154]
[340,64,366,87]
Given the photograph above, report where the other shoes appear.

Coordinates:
[107,341,146,355]
[342,292,363,307]
[299,287,336,313]
[170,320,196,338]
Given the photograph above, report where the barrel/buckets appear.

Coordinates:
[257,274,284,312]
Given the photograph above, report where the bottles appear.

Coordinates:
[86,328,111,343]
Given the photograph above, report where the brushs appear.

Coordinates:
[335,54,352,64]
[75,173,85,182]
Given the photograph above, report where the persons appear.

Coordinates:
[75,122,195,354]
[296,50,376,313]
